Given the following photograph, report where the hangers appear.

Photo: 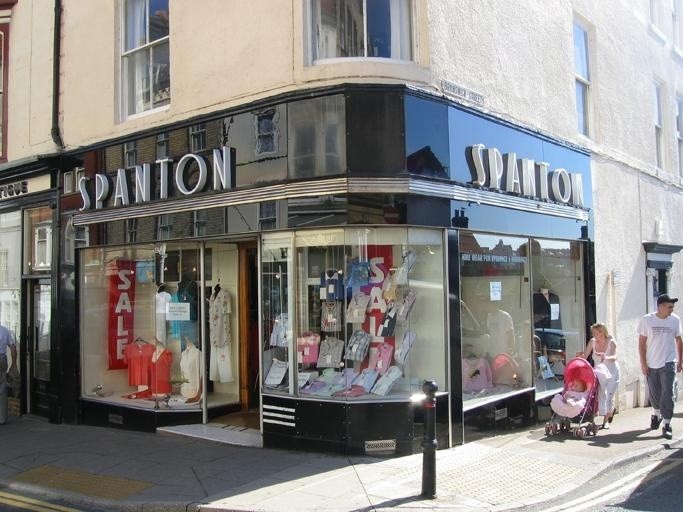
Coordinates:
[133,338,146,344]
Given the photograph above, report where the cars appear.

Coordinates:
[458,298,492,356]
[530,326,579,382]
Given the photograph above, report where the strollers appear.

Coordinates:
[543,355,603,440]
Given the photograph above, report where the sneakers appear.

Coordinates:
[662,423,672,440]
[599,408,616,429]
[651,415,662,430]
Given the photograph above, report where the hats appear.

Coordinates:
[657,294,678,304]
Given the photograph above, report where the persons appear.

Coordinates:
[149,343,173,398]
[0,326,18,426]
[561,379,587,406]
[575,322,621,428]
[533,287,567,367]
[485,302,515,353]
[150,285,172,347]
[636,293,683,440]
[209,283,235,383]
[516,320,542,358]
[179,343,201,399]
[176,279,198,353]
